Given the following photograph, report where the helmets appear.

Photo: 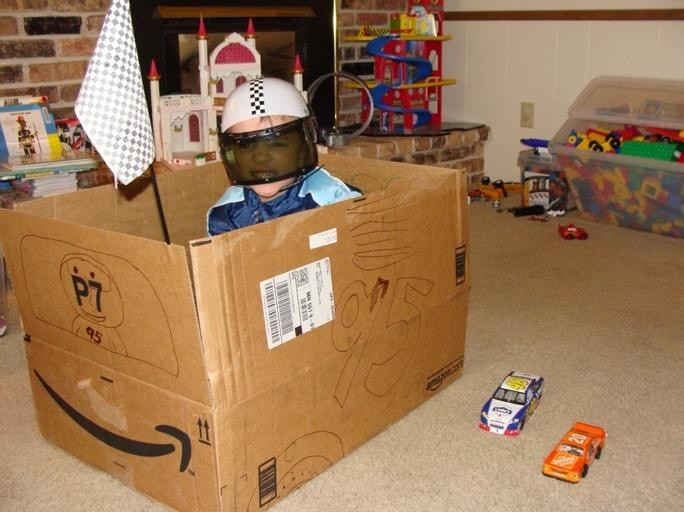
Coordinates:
[217,77,319,145]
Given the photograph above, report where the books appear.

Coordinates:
[466,96,683,240]
[0,90,96,205]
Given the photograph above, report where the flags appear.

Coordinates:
[71,0,158,190]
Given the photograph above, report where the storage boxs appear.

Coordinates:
[517,149,577,215]
[547,75,684,240]
[1,153,471,512]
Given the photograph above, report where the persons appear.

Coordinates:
[202,76,364,236]
